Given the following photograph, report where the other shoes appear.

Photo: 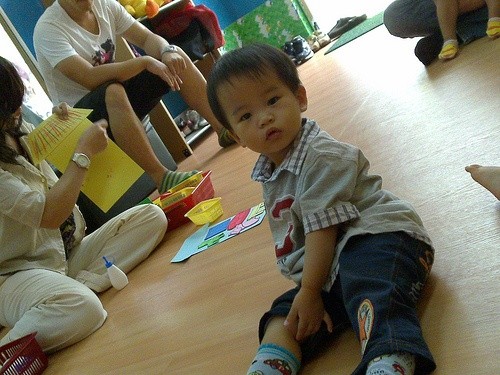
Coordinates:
[326,14,367,39]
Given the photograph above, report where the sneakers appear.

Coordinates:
[280,34,314,67]
[305,29,332,52]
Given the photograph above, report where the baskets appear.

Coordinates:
[184,196,224,226]
[147,168,216,234]
[0,331,50,375]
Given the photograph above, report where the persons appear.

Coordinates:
[384,0,500,66]
[34,0,238,194]
[465,164,500,201]
[0,56,167,359]
[206,42,435,375]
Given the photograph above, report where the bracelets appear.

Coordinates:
[161,45,177,56]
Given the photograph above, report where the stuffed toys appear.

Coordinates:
[120,0,171,19]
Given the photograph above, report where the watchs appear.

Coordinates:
[71,153,91,170]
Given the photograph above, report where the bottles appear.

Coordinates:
[102,255,129,291]
